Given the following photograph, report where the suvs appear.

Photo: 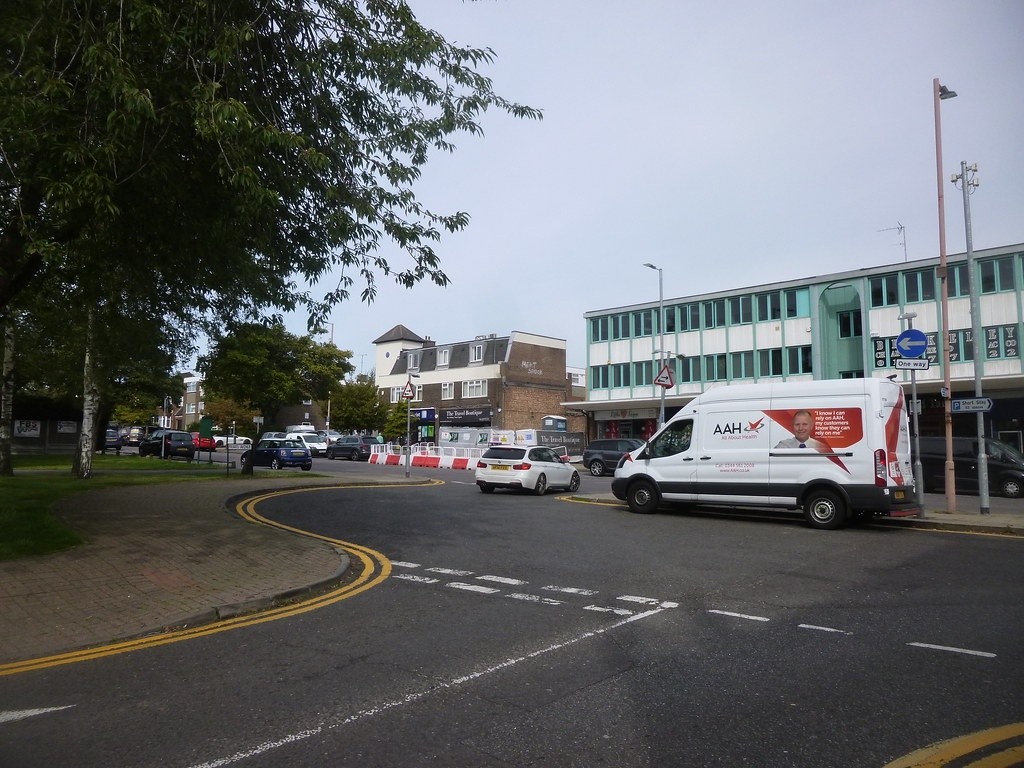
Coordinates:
[582,439,668,477]
[474,444,581,497]
[325,436,381,461]
[139,430,196,461]
[118,425,169,447]
[910,436,1024,499]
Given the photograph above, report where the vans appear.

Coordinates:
[611,378,916,528]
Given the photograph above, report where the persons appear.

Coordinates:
[774,411,824,448]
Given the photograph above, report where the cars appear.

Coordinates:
[240,425,328,471]
[190,432,217,451]
[316,430,344,444]
[212,434,253,447]
[105,430,124,451]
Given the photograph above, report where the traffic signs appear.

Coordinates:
[952,397,993,412]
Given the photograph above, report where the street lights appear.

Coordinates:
[643,263,687,435]
[163,396,172,430]
[897,312,926,519]
[326,391,331,447]
[932,77,960,515]
[405,373,420,479]
